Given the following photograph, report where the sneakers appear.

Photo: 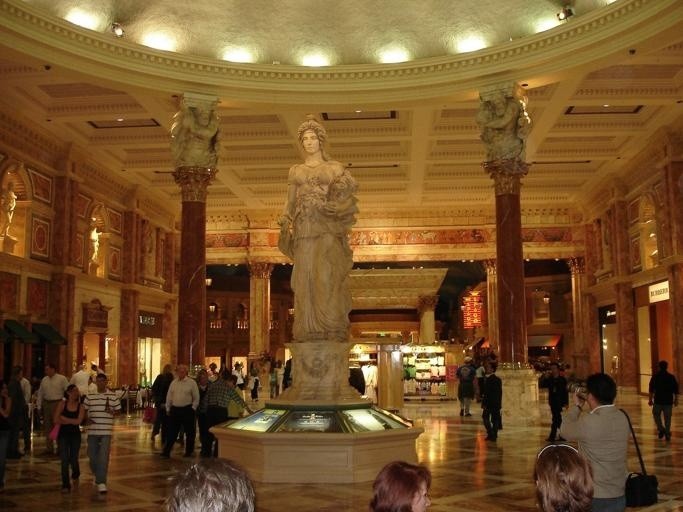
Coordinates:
[60,477,79,493]
[93,478,107,492]
[159,451,194,459]
[460,409,470,416]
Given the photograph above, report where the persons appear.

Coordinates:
[539,363,570,442]
[456,355,503,441]
[369,460,431,512]
[161,457,256,512]
[276,119,360,342]
[147,359,291,463]
[647,360,679,443]
[559,372,632,512]
[0,360,122,494]
[534,443,595,512]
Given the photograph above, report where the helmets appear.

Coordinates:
[463,357,472,363]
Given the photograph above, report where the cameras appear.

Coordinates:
[567,381,588,400]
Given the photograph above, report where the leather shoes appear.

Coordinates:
[545,436,569,443]
[484,434,497,441]
[658,426,672,441]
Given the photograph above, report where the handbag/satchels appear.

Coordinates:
[50,422,59,440]
[624,472,659,508]
[142,405,157,423]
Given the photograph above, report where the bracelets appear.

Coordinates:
[575,404,583,411]
